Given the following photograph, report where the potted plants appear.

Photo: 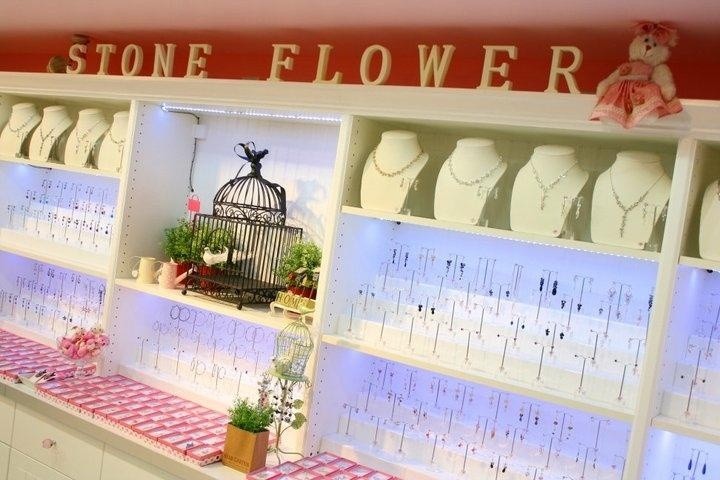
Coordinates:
[221,395,271,473]
[270,238,317,298]
[165,220,198,287]
[190,224,235,290]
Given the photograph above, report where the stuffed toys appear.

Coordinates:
[588,21,683,129]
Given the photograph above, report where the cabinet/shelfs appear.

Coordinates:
[299,109,694,480]
[0,383,220,480]
[96,96,343,457]
[628,133,720,480]
[1,87,134,374]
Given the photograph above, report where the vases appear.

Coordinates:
[76,361,97,381]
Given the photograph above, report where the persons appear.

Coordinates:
[529,160,580,211]
[609,166,666,238]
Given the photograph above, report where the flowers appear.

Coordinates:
[56,321,109,360]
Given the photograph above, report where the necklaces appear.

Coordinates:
[75,118,104,154]
[109,129,126,174]
[373,148,425,177]
[8,110,38,141]
[448,153,504,186]
[39,114,70,156]
